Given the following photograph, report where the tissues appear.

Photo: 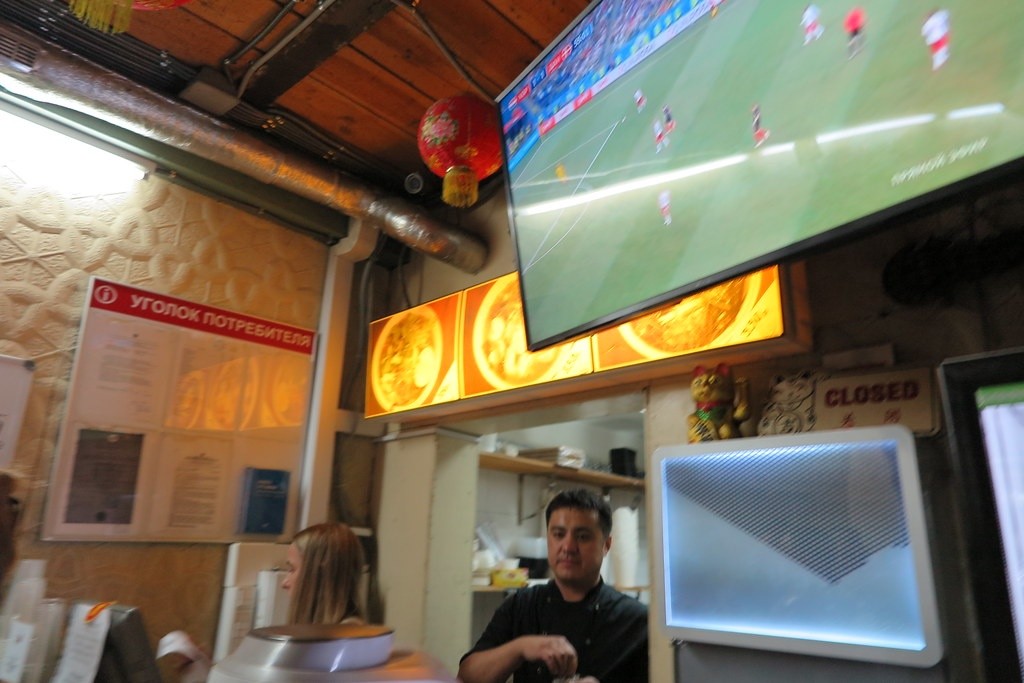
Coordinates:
[490,557,529,590]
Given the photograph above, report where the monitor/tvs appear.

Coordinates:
[68,601,163,683]
[497,0,1024,353]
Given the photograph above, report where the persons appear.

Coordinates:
[842,7,865,58]
[282,523,365,626]
[752,103,770,147]
[658,189,672,225]
[801,3,826,45]
[653,105,676,154]
[921,7,952,70]
[0,470,24,594]
[501,0,681,155]
[555,163,567,185]
[459,489,649,683]
[633,89,646,112]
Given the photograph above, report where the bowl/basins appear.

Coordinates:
[619,270,761,359]
[472,273,572,389]
[371,306,443,413]
[271,357,303,423]
[205,357,260,431]
[171,369,205,427]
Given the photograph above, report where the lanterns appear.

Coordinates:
[418,92,503,208]
